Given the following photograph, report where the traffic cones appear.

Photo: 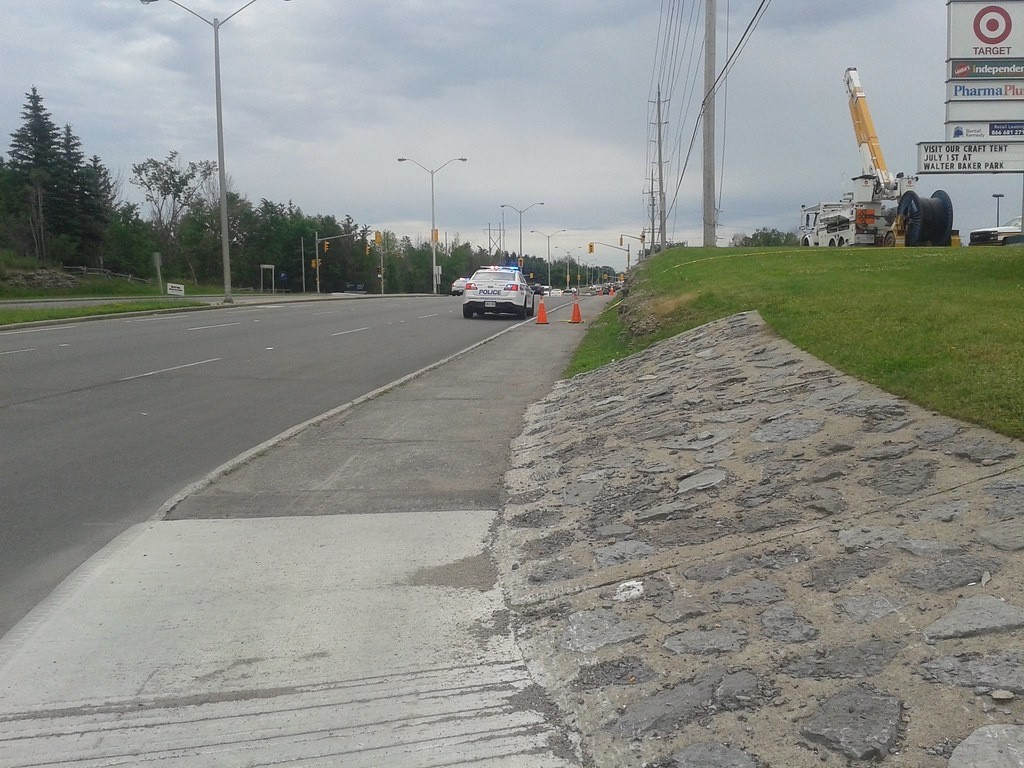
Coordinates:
[568,295,584,324]
[534,296,550,324]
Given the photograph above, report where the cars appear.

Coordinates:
[463,265,535,320]
[451,277,471,296]
[527,281,622,297]
[968,215,1023,246]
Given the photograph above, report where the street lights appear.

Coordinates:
[992,194,1005,227]
[499,202,545,272]
[141,0,290,305]
[529,229,608,290]
[397,157,467,294]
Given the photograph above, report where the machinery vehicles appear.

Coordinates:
[798,66,905,248]
